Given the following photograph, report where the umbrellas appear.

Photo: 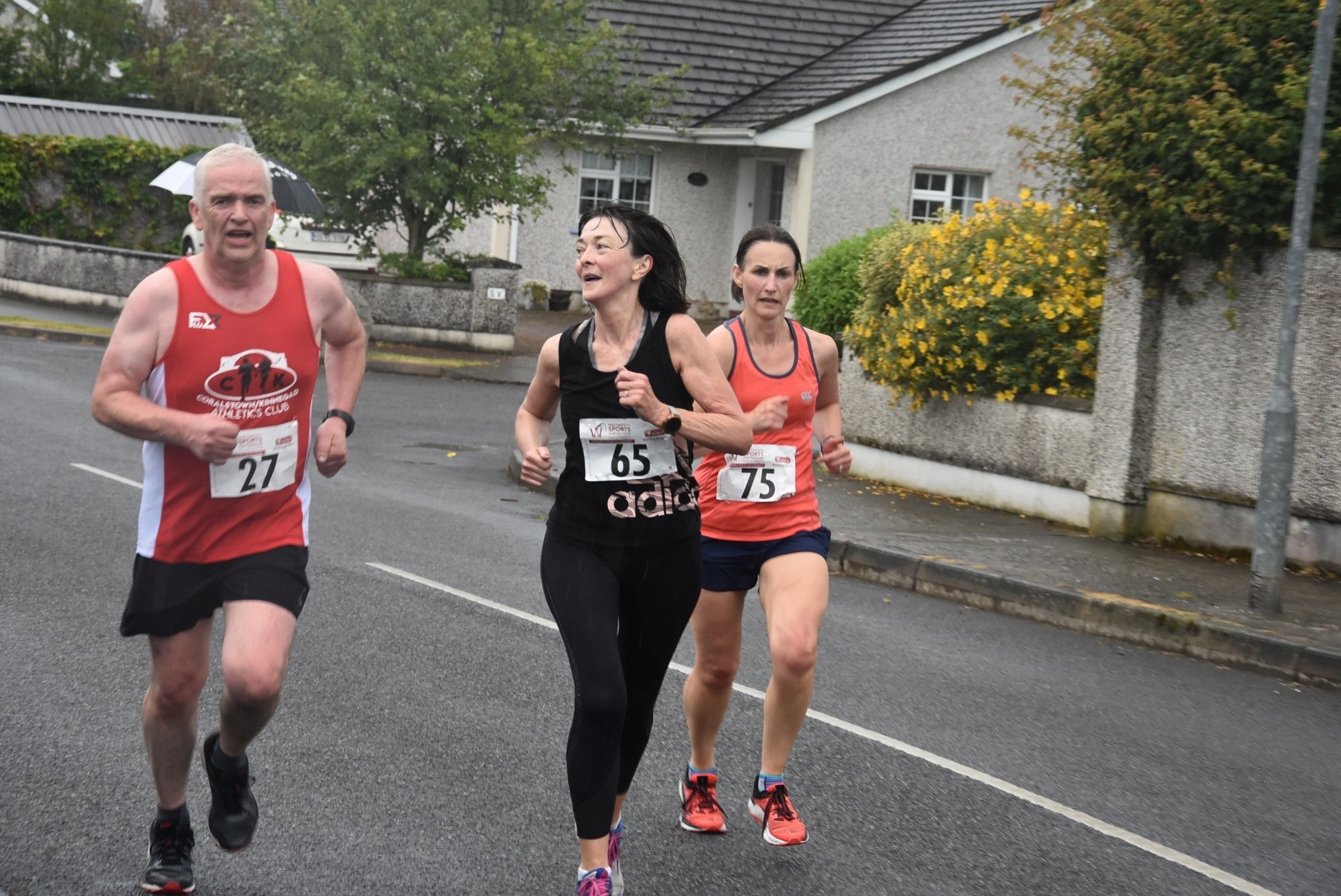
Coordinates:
[148,141,325,217]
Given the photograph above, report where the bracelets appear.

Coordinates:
[820,434,845,453]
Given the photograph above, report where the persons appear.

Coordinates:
[679,221,853,846]
[515,200,750,896]
[91,143,368,895]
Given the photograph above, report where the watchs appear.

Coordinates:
[322,408,355,437]
[661,406,682,435]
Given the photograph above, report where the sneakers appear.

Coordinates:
[141,818,195,895]
[200,732,259,850]
[576,809,624,896]
[748,772,809,846]
[678,759,728,833]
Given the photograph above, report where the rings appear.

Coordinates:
[839,465,844,474]
[624,391,628,398]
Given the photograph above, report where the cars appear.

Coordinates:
[189,175,382,275]
